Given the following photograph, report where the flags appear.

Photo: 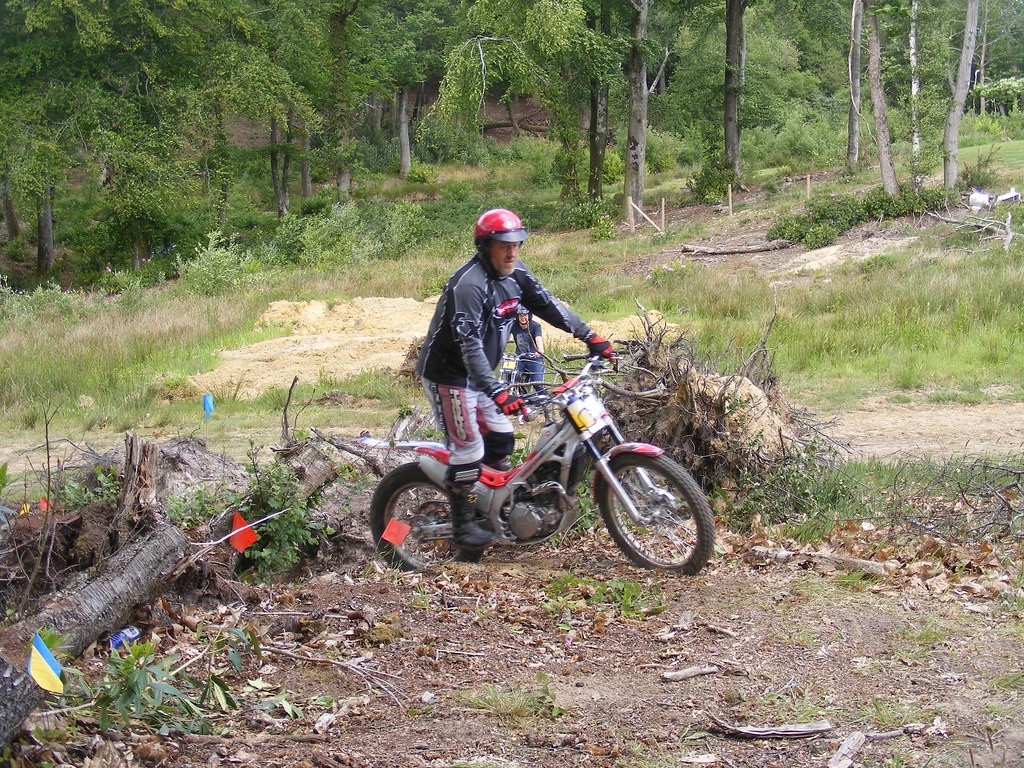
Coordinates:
[229,513,258,553]
[27,632,64,694]
[382,519,412,546]
[203,395,214,419]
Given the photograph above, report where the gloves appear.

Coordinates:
[585,334,616,364]
[490,388,528,418]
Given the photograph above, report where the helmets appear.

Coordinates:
[475,208,529,245]
[517,306,529,313]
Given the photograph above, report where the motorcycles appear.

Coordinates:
[368,347,713,575]
[501,341,537,403]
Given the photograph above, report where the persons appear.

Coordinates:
[510,304,555,426]
[415,209,616,551]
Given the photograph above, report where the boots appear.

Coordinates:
[446,485,501,550]
